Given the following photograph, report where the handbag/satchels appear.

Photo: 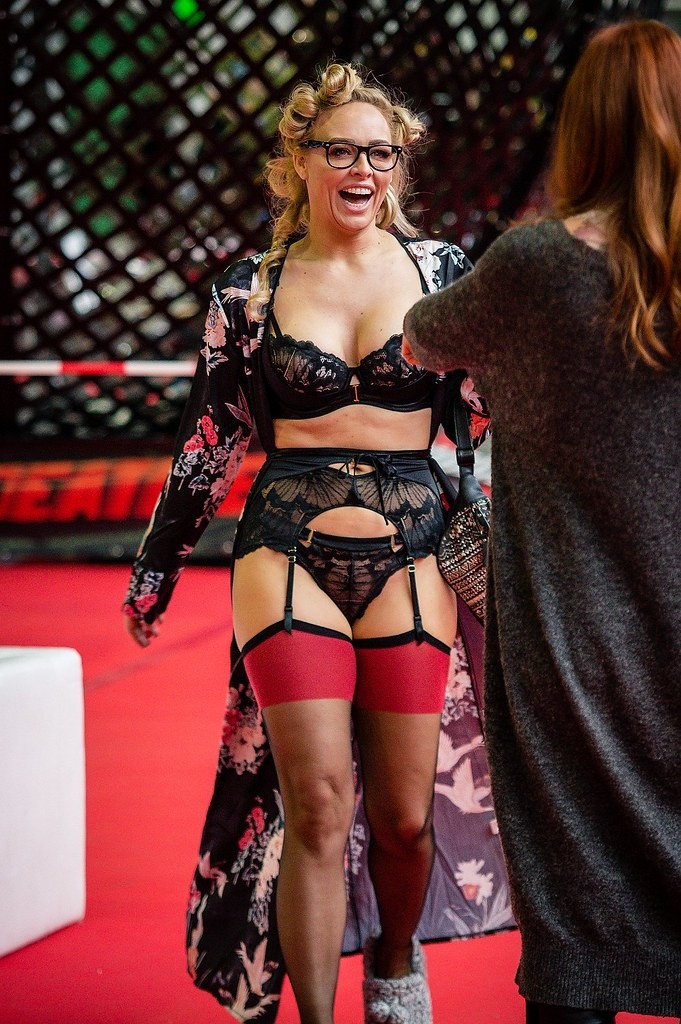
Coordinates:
[435,483,490,628]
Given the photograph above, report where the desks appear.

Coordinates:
[0,644,87,961]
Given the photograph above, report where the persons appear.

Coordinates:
[119,59,484,1024]
[402,16,681,1024]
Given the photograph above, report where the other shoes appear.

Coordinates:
[363,934,434,1023]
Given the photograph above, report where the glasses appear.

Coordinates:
[299,140,402,172]
